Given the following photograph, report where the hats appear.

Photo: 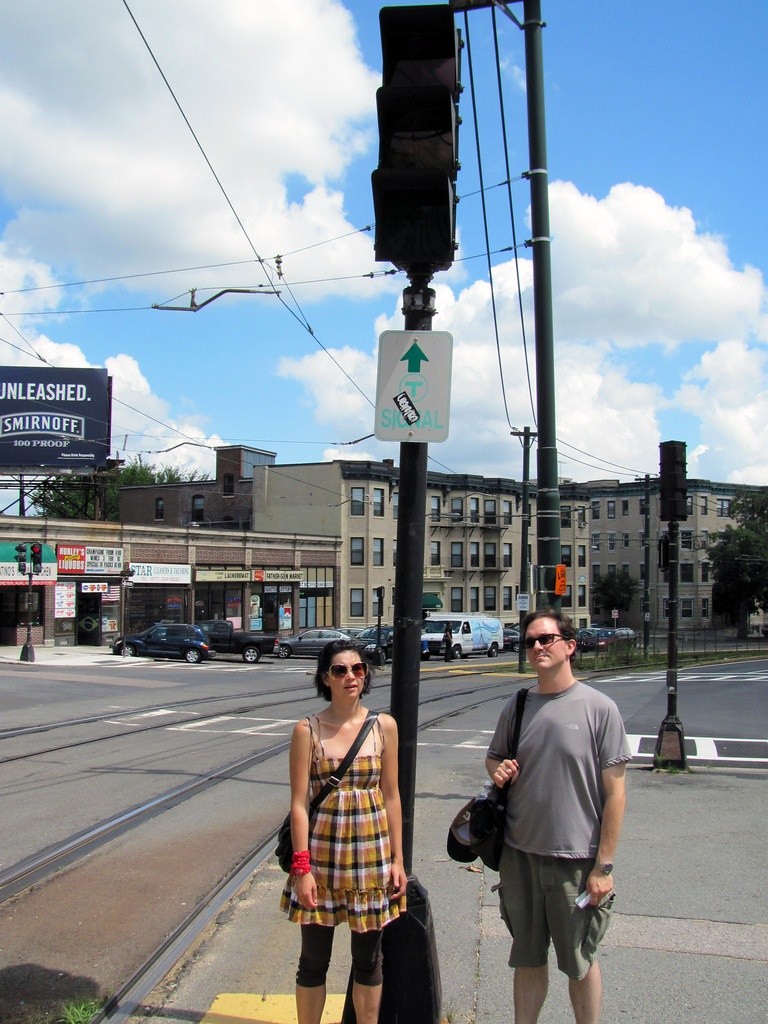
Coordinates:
[447,799,478,863]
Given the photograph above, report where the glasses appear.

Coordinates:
[327,662,369,681]
[521,633,570,649]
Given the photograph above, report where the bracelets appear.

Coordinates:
[290,850,311,876]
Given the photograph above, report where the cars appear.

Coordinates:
[278,629,355,659]
[113,621,215,663]
[502,623,520,652]
[573,627,596,648]
[355,623,393,660]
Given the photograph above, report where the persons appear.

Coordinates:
[280,639,407,1024]
[444,622,454,662]
[484,609,632,1024]
[456,624,470,634]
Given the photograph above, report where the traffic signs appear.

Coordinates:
[122,580,134,587]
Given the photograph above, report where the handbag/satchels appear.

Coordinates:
[275,805,294,874]
[471,688,530,873]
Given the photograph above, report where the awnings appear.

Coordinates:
[422,593,443,609]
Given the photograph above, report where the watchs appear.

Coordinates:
[594,862,614,874]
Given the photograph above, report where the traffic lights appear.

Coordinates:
[30,543,42,572]
[371,4,465,263]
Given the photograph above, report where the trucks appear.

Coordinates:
[421,610,504,659]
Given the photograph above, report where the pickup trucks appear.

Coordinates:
[197,620,281,664]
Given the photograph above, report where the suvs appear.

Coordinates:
[582,628,637,652]
